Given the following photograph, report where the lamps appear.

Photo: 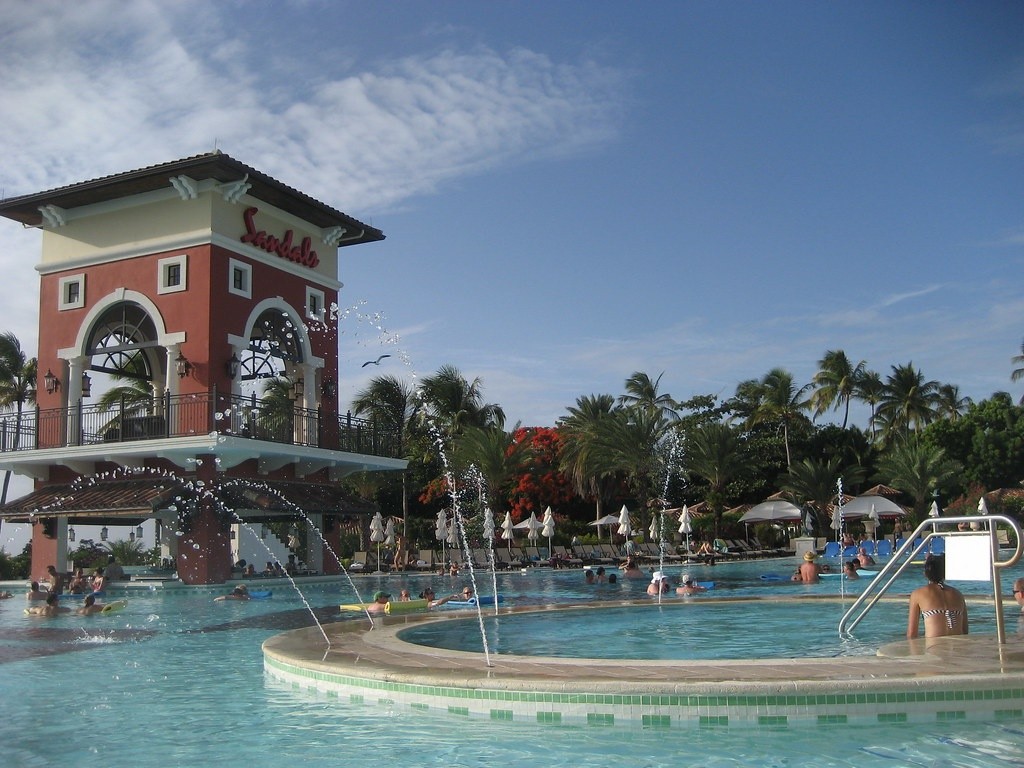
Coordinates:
[225,352,241,380]
[137,522,143,538]
[287,378,303,402]
[100,525,108,541]
[44,368,58,394]
[81,371,92,397]
[69,525,75,542]
[174,350,192,379]
[130,526,135,542]
[325,377,335,400]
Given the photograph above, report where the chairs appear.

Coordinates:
[349,537,796,576]
[995,529,1010,545]
[816,530,945,557]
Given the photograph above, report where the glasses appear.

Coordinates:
[823,568,829,570]
[464,591,472,594]
[1013,590,1019,594]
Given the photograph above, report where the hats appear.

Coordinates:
[683,574,694,584]
[651,570,668,584]
[374,592,391,601]
[803,552,817,561]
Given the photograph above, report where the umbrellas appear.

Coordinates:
[287,534,301,552]
[617,505,630,556]
[978,496,988,531]
[435,509,448,568]
[482,507,495,538]
[830,495,905,547]
[588,514,619,544]
[369,512,395,570]
[929,500,939,531]
[737,498,814,536]
[446,519,458,548]
[501,505,555,557]
[678,504,693,561]
[649,516,657,543]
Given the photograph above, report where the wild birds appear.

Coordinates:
[362,355,391,367]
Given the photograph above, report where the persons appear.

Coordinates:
[907,553,968,636]
[1012,577,1024,611]
[383,532,459,575]
[958,522,969,531]
[790,518,912,581]
[585,556,707,594]
[213,554,296,600]
[367,587,473,610]
[690,540,715,555]
[26,556,125,615]
